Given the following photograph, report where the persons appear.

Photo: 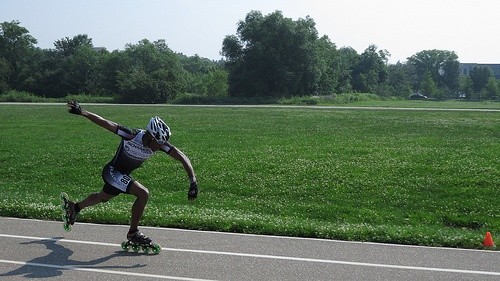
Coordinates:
[60,100,198,253]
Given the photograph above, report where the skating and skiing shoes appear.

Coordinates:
[121,227,161,253]
[60,192,81,231]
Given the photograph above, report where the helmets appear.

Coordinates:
[147,115,171,144]
